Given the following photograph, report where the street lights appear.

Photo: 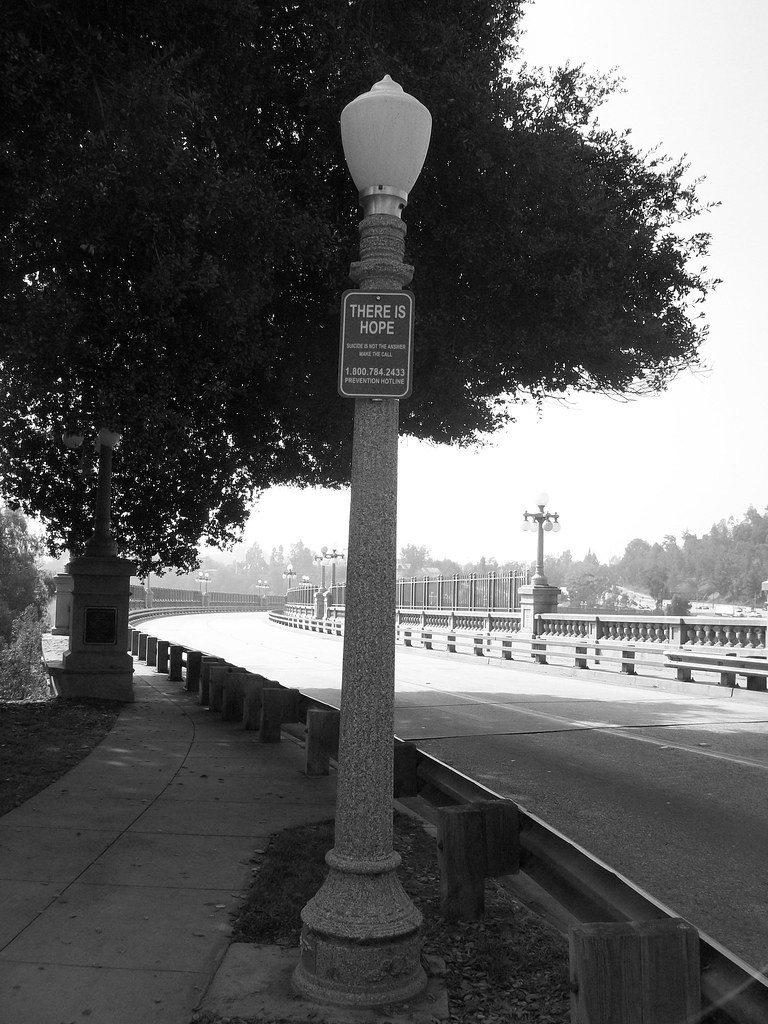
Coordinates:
[61,427,122,557]
[521,492,561,585]
[313,546,347,594]
[256,580,270,598]
[283,564,297,589]
[290,68,440,1007]
[302,575,311,583]
[194,572,212,596]
